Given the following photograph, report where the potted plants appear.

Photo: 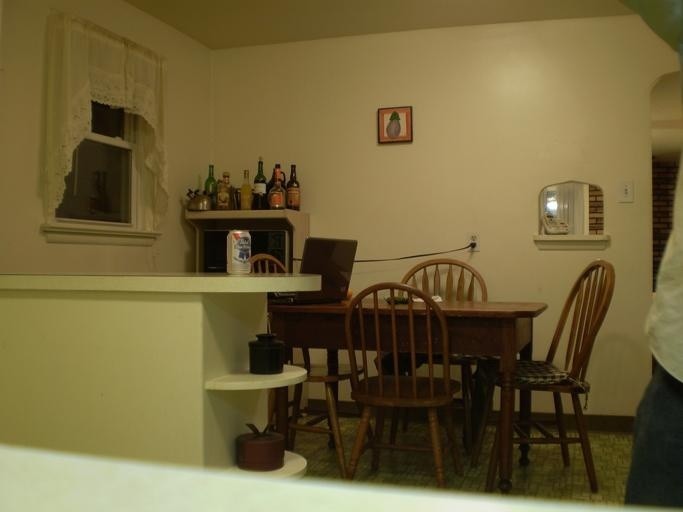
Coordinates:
[233,420,286,472]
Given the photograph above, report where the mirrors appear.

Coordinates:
[538,180,605,235]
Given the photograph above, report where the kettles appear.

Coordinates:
[187,188,211,210]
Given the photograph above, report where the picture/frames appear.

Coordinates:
[377,105,413,144]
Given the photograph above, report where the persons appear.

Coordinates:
[627,147,682,507]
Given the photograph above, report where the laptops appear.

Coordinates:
[272,238,358,306]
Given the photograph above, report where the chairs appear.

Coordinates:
[380,257,488,454]
[344,281,464,488]
[471,259,615,495]
[238,253,376,484]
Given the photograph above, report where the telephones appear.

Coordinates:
[541,211,569,234]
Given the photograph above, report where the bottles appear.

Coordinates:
[216,171,234,210]
[205,165,217,210]
[254,160,267,209]
[226,230,251,276]
[268,170,286,210]
[271,164,285,185]
[241,170,252,210]
[287,164,301,210]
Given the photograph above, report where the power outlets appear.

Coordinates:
[468,232,481,251]
[615,179,636,204]
